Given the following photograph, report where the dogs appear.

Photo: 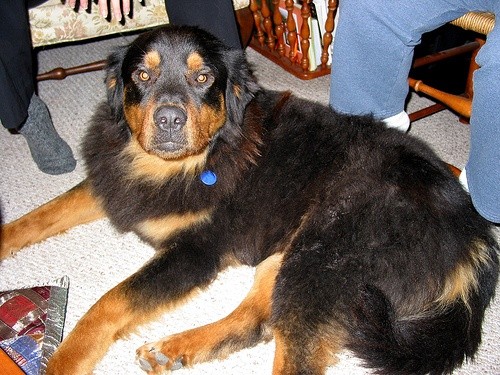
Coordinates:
[1,24,500,375]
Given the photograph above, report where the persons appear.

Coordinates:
[0,0,246,176]
[331,0,500,230]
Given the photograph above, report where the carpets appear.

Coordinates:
[0,31,500,375]
